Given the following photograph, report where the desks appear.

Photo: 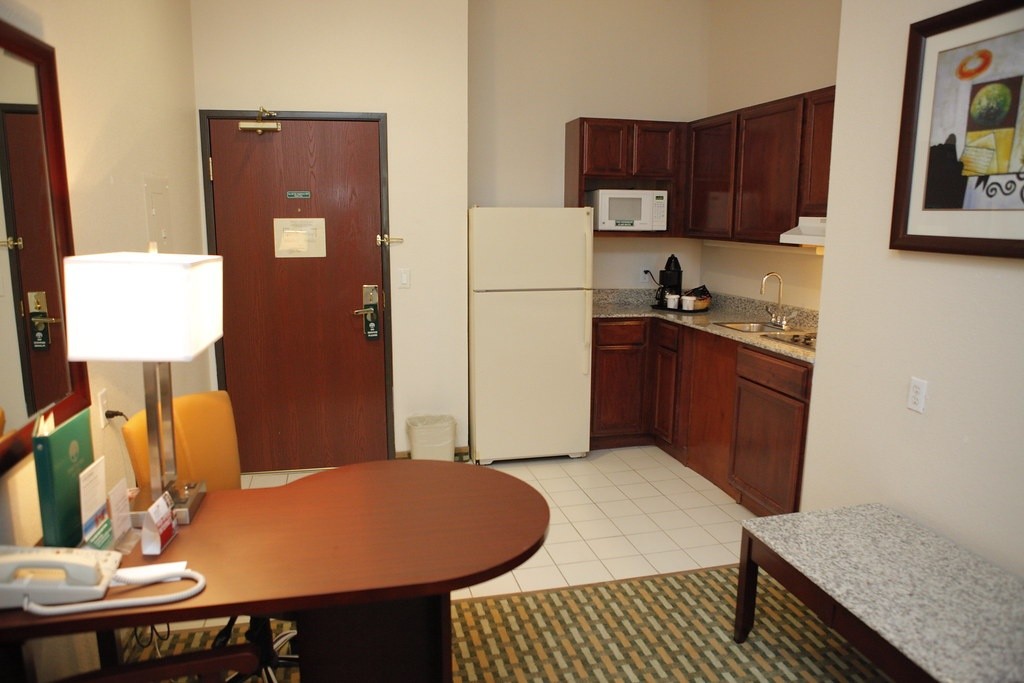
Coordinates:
[731,500,1024,683]
[0,458,552,682]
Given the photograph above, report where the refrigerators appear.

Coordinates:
[468,207,594,466]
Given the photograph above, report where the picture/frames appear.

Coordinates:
[888,0,1024,256]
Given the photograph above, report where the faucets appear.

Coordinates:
[760,271,799,328]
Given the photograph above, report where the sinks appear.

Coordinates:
[713,321,787,332]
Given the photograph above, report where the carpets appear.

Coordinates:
[121,557,894,682]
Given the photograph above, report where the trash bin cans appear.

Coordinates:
[406,414,455,461]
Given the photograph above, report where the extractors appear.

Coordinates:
[779,217,827,245]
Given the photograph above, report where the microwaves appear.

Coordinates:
[586,189,668,231]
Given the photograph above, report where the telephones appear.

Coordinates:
[0,545,122,608]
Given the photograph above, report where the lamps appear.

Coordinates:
[61,252,232,530]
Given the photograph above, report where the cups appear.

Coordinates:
[681,296,696,310]
[665,294,680,309]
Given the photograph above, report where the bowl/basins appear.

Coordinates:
[694,297,711,309]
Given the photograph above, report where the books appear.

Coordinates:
[32,408,94,548]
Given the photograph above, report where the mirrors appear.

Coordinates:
[0,19,92,477]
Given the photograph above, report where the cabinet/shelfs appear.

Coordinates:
[590,315,653,455]
[654,315,688,467]
[684,95,806,247]
[797,88,837,216]
[728,343,813,517]
[566,116,685,180]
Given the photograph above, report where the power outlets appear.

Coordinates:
[907,377,928,415]
[640,267,649,282]
[97,389,114,428]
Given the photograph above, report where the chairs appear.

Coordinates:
[125,390,244,508]
